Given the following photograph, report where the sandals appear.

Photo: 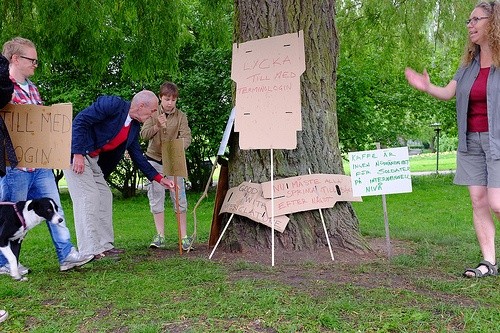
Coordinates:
[462,260,497,278]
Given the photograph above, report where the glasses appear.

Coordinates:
[466,16,490,25]
[11,54,38,65]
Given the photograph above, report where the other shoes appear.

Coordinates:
[92,253,121,263]
[0,310,7,323]
[103,248,125,255]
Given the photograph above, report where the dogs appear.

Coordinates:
[0,198,63,282]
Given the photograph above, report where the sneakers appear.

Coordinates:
[149,233,165,247]
[59,247,94,271]
[179,235,194,250]
[0,261,31,275]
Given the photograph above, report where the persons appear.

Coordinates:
[405,0,500,279]
[62,89,180,261]
[0,37,96,324]
[140,82,194,252]
